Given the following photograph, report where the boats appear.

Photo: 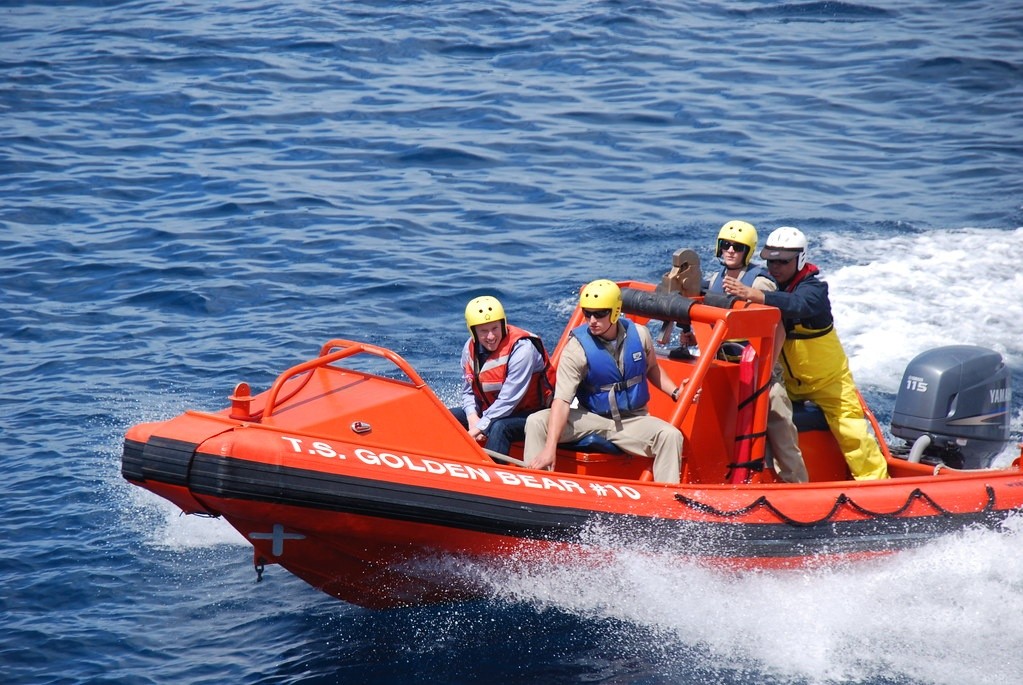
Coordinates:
[119,247,1023,612]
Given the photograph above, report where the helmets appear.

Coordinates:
[580,279,622,325]
[760,225,806,272]
[464,295,509,343]
[716,220,758,266]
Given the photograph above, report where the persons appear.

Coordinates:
[524,278,701,484]
[678,218,809,483]
[447,295,557,466]
[721,226,891,481]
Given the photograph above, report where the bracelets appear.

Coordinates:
[671,387,680,401]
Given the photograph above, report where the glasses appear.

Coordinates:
[720,240,747,253]
[583,308,612,319]
[768,258,795,264]
